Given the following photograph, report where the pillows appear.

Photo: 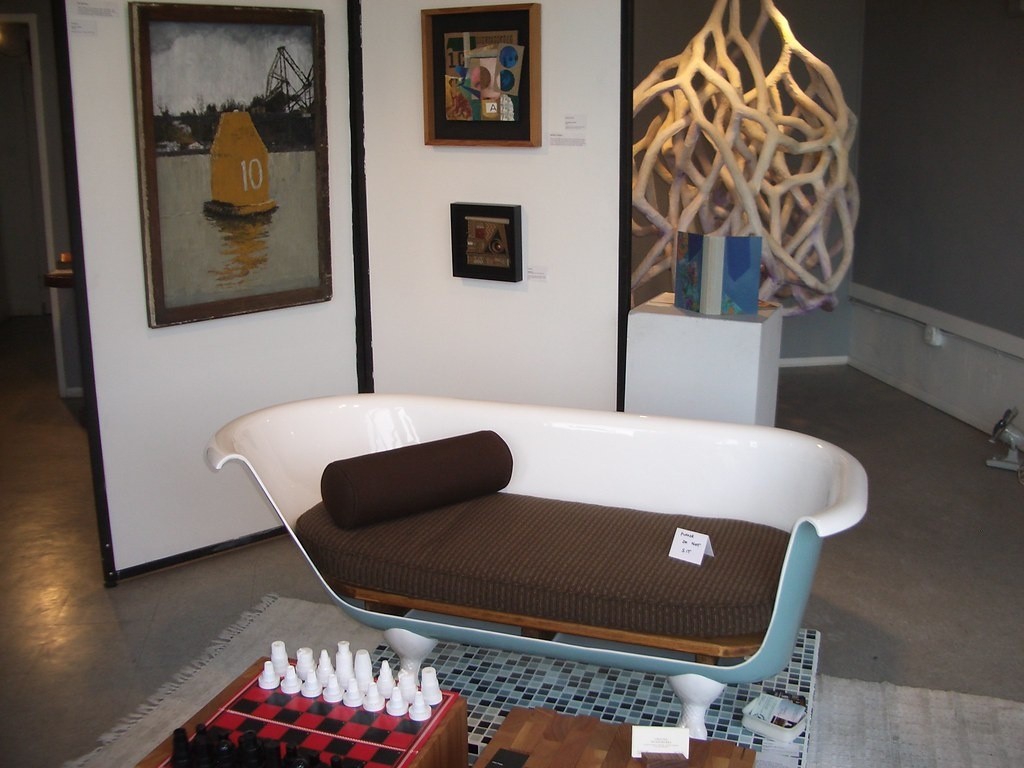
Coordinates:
[321,430,513,529]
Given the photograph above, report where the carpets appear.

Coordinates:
[64,591,1024,767]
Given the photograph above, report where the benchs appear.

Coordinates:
[203,394,869,740]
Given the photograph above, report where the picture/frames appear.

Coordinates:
[421,3,542,147]
[450,202,523,283]
[129,1,332,329]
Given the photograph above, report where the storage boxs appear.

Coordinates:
[742,698,808,744]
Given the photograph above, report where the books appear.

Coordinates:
[674,231,780,316]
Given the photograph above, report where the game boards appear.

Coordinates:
[157,660,461,766]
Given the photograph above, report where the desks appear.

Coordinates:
[135,656,468,768]
[471,707,757,768]
